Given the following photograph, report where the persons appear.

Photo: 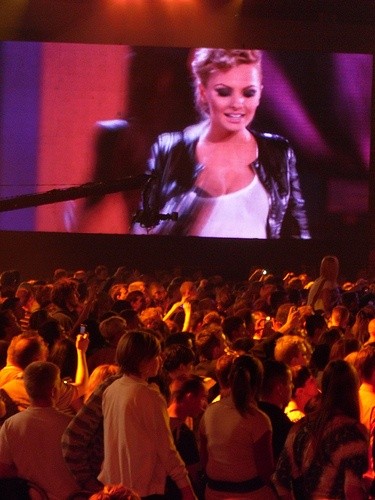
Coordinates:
[0,255,375,500]
[131,49,310,239]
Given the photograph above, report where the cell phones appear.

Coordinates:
[80,324,87,336]
